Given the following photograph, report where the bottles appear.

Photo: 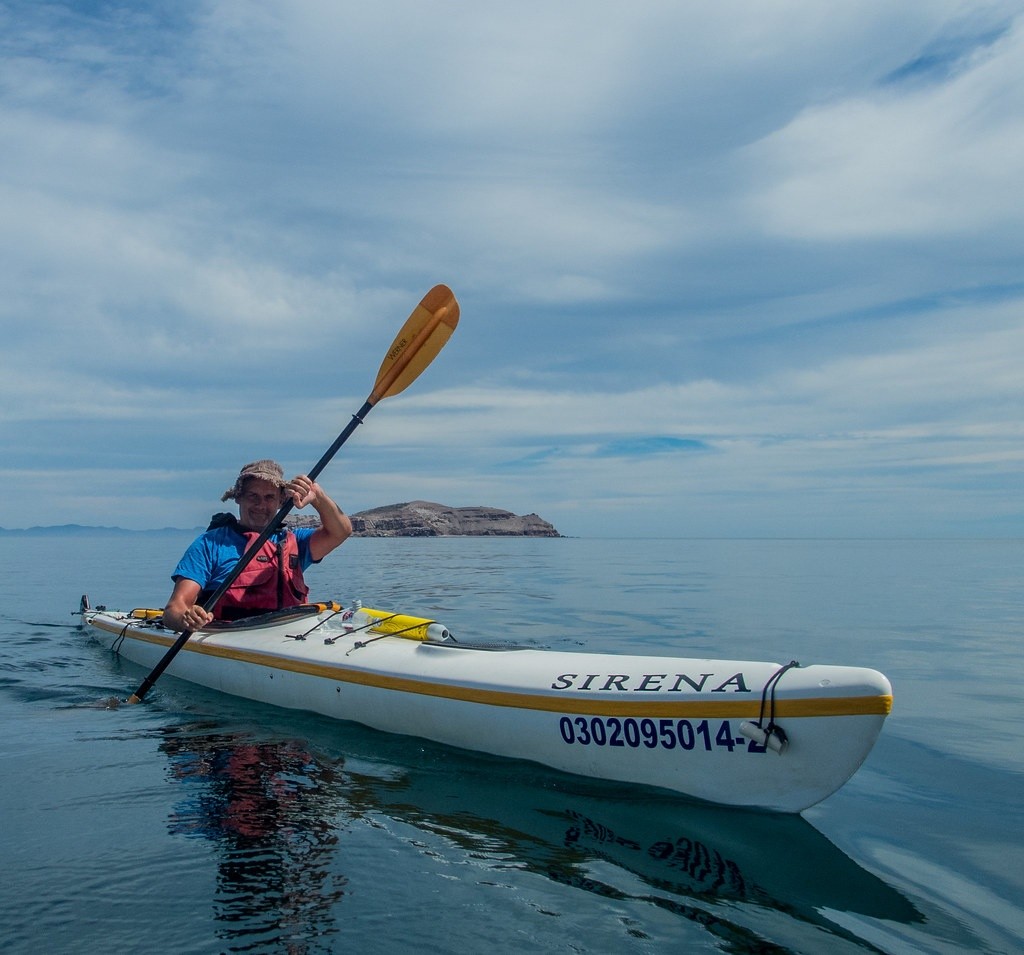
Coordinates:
[318,610,381,633]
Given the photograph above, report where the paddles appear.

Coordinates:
[125,284,459,707]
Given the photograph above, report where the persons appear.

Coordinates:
[162,460,352,632]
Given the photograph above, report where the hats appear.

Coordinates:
[221,459,290,509]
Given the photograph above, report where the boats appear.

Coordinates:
[135,666,1024,955]
[75,594,892,816]
